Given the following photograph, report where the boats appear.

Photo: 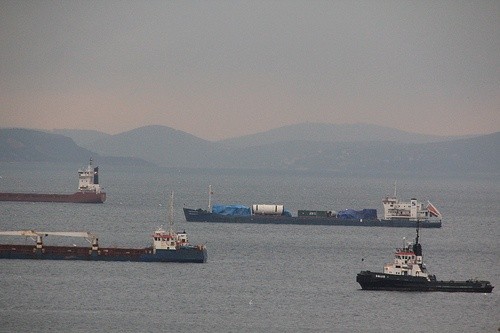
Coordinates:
[183,185,443,228]
[0,192,209,264]
[0,158,106,204]
[356,220,494,293]
